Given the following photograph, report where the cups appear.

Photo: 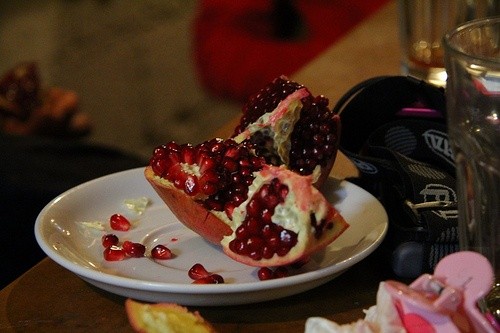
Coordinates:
[397,0,499,88]
[441,14,500,319]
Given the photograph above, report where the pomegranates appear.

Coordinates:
[102,74,351,284]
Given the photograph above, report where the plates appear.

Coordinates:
[33,164,388,306]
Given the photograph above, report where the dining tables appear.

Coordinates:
[0,0,499,333]
